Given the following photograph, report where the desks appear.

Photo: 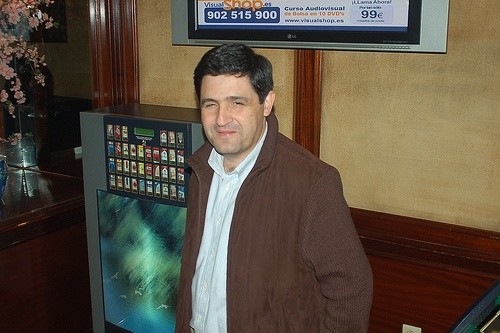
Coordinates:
[0,165,93,333]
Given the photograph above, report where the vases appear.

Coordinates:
[0,156,7,201]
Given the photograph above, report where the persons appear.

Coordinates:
[174,42,374,333]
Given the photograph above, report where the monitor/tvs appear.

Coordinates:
[171,0,451,55]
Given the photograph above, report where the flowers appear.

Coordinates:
[0,0,58,146]
